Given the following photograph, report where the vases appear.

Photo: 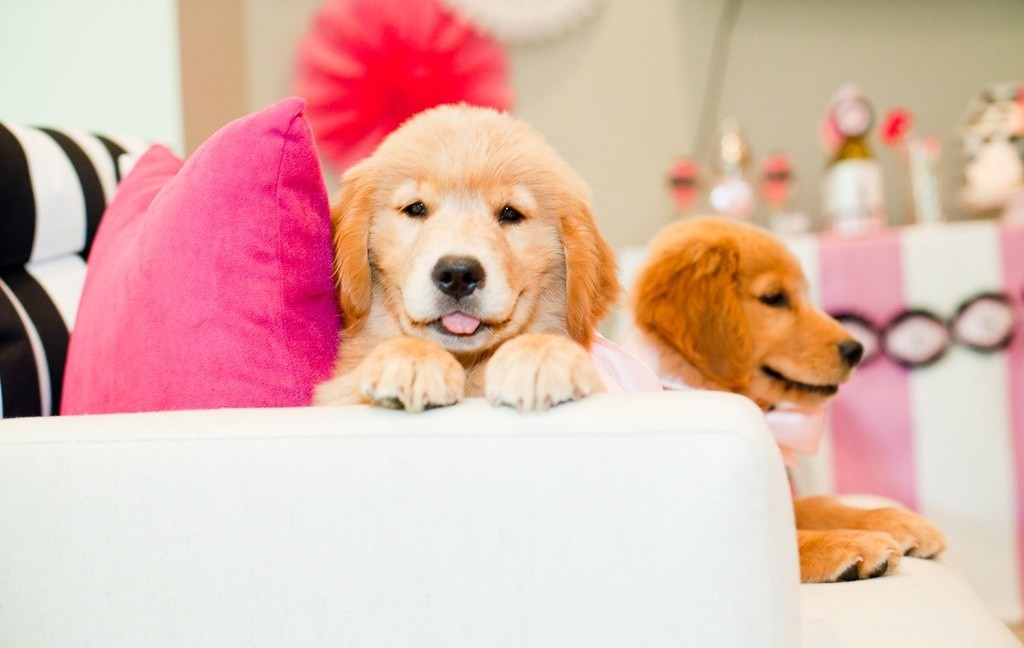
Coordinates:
[911,139,945,227]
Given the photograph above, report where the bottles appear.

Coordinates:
[707,124,763,227]
[826,125,889,230]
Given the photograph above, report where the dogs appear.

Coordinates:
[307,103,624,412]
[596,214,952,584]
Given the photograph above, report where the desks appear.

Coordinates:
[620,217,1024,625]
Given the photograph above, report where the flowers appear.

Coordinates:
[881,111,920,142]
[960,79,1023,206]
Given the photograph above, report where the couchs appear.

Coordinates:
[0,127,1023,648]
